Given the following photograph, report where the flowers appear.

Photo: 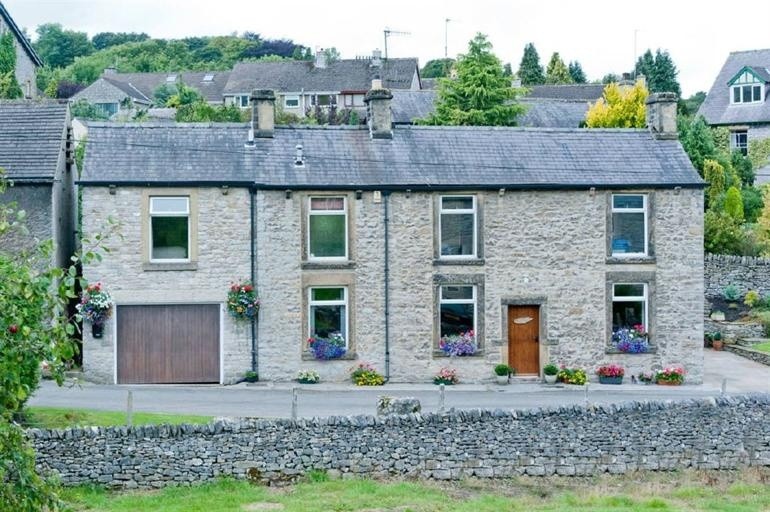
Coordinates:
[559,363,687,386]
[225,280,260,321]
[307,331,347,361]
[299,363,461,385]
[612,324,649,354]
[439,330,476,355]
[75,283,114,325]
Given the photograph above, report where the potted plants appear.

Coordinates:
[542,364,558,384]
[495,364,509,385]
